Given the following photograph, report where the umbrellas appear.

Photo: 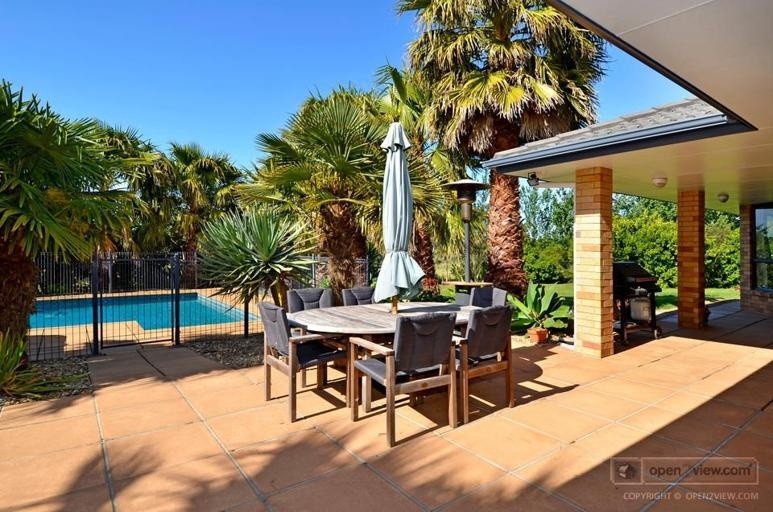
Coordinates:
[374,122,426,315]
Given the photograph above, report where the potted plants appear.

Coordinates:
[507,279,569,343]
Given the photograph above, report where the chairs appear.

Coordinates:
[258,286,514,447]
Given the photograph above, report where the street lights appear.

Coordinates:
[440,179,494,282]
